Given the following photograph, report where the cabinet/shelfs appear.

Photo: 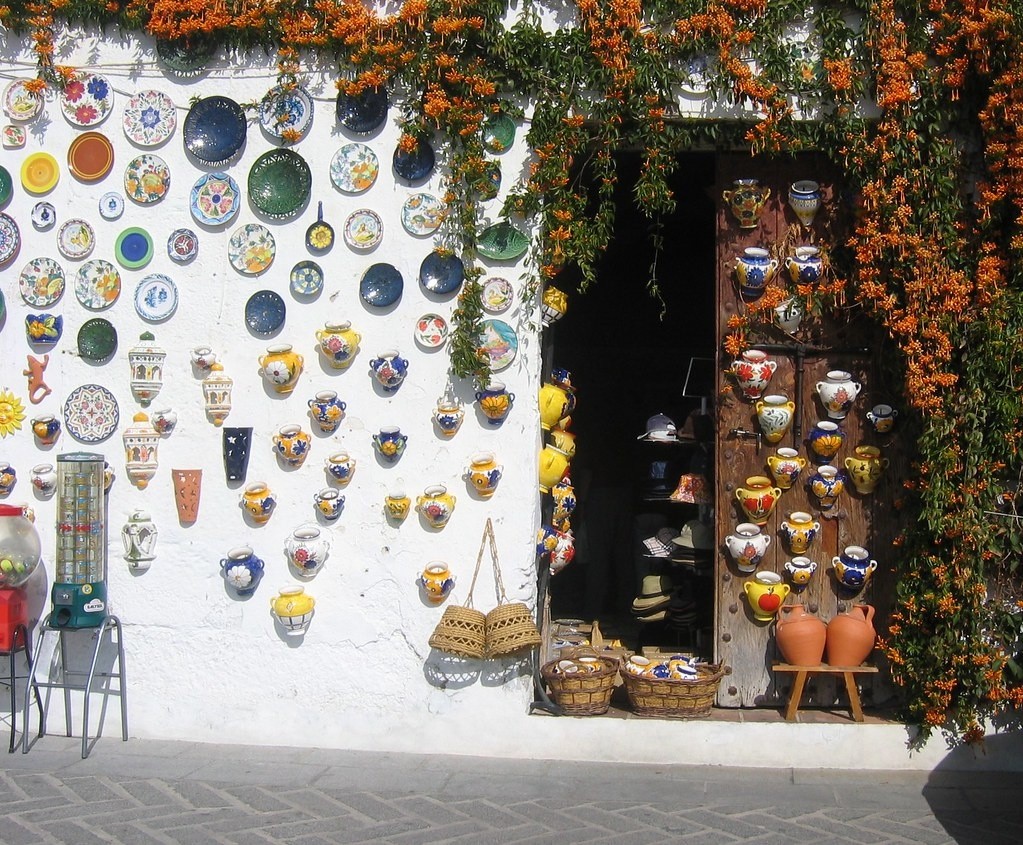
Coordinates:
[637,436,714,649]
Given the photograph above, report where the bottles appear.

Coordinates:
[121,509,158,571]
[30,414,61,446]
[29,464,57,496]
[539,370,576,574]
[0,504,41,588]
[774,605,826,667]
[0,462,16,495]
[826,604,876,667]
[220,319,516,639]
[722,178,900,623]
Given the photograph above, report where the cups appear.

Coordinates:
[551,655,700,680]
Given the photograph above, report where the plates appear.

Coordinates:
[64,384,119,443]
[0,69,531,331]
[77,320,118,360]
[475,319,518,371]
[414,314,448,349]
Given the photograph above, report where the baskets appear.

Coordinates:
[619,656,732,719]
[541,645,619,716]
[485,602,542,660]
[428,605,486,661]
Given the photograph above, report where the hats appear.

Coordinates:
[630,408,714,631]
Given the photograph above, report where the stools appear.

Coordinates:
[771,661,879,721]
